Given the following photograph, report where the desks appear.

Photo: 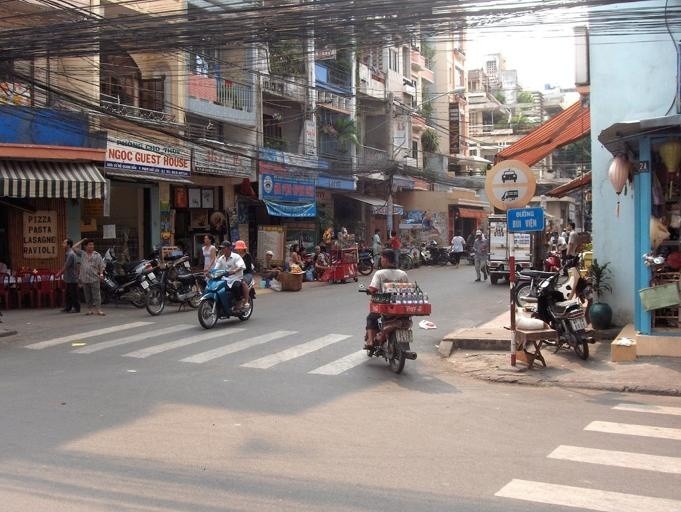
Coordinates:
[504,325,558,370]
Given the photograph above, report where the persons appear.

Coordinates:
[204,241,246,309]
[234,239,254,306]
[364,248,409,352]
[545,223,578,276]
[262,229,489,287]
[72,238,105,315]
[202,234,216,283]
[55,238,81,313]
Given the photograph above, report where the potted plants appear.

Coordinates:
[583,259,614,329]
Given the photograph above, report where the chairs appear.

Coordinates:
[0,267,67,310]
[652,272,681,328]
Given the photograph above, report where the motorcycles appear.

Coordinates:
[348,240,457,277]
[514,252,590,312]
[81,245,257,328]
[357,286,418,374]
[530,266,590,360]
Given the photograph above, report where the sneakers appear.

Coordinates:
[58,306,106,316]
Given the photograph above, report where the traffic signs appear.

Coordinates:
[508,207,544,234]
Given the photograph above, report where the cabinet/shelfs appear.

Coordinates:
[331,240,359,284]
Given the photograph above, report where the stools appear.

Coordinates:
[260,280,272,288]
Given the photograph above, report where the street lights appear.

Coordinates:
[386,85,467,242]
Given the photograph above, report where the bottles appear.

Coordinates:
[390,290,429,305]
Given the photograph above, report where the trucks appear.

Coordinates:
[486,215,559,285]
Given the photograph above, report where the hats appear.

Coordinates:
[218,240,233,248]
[234,240,249,250]
[476,230,483,236]
